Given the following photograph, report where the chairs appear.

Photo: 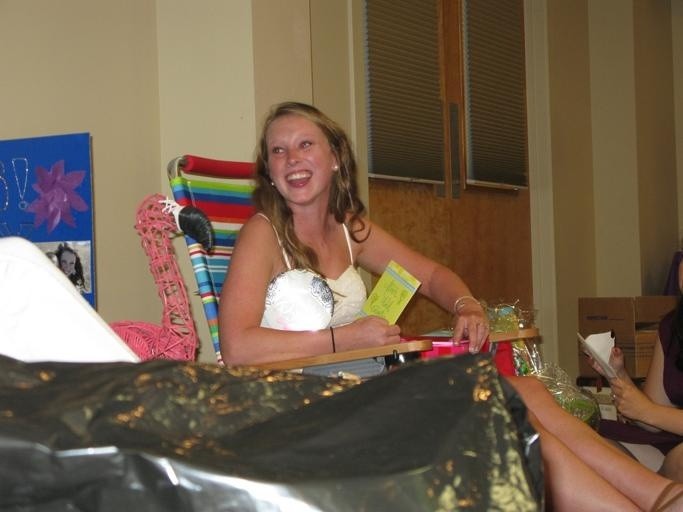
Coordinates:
[167,155,539,378]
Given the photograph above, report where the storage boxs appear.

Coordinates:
[577,295,677,380]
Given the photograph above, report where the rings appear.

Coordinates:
[611,393,616,399]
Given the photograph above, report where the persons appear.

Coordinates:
[55,246,84,289]
[215,102,682,511]
[582,254,682,484]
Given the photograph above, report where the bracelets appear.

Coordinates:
[453,295,480,318]
[328,326,337,353]
